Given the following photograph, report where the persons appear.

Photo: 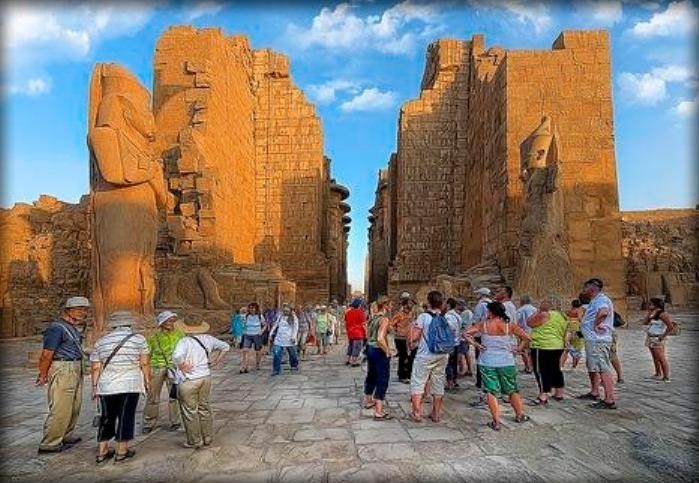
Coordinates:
[34,296,90,454]
[171,313,230,448]
[230,279,625,431]
[142,309,185,434]
[643,298,674,384]
[89,312,151,466]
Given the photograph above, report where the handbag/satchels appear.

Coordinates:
[613,313,625,327]
[666,323,680,336]
[169,383,177,400]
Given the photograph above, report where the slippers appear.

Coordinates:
[529,398,547,406]
[365,400,376,409]
[375,414,392,421]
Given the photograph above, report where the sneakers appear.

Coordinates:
[657,378,670,384]
[183,436,212,448]
[143,426,151,433]
[168,424,180,431]
[114,449,135,464]
[96,448,114,465]
[38,435,80,454]
[93,416,101,427]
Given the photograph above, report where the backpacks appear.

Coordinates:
[420,309,454,354]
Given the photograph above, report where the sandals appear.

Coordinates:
[487,422,499,431]
[578,393,600,400]
[516,416,529,423]
[590,401,616,409]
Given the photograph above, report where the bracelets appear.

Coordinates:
[92,385,96,388]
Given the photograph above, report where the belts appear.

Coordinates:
[53,358,81,362]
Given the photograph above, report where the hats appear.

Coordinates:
[61,296,91,310]
[155,311,177,328]
[474,288,492,296]
[105,312,133,329]
[173,313,211,335]
